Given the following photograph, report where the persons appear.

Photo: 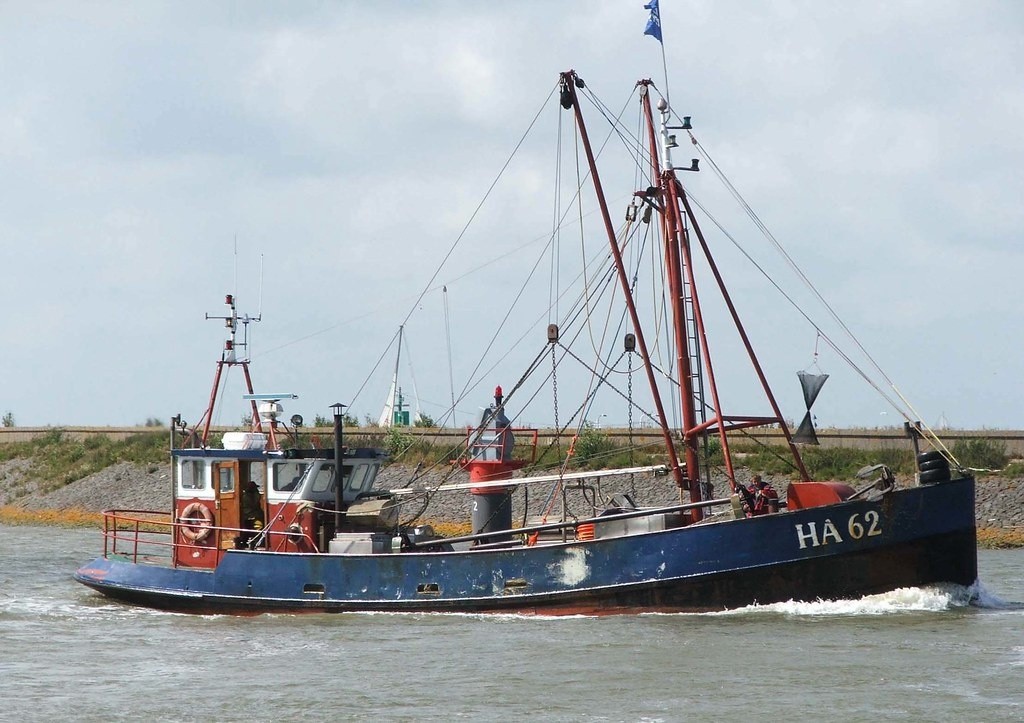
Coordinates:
[748,475,769,490]
[242,481,265,547]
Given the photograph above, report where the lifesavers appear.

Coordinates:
[916,448,952,486]
[180,502,214,541]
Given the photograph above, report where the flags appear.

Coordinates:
[643,0,662,42]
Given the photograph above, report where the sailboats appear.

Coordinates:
[72,76,981,618]
